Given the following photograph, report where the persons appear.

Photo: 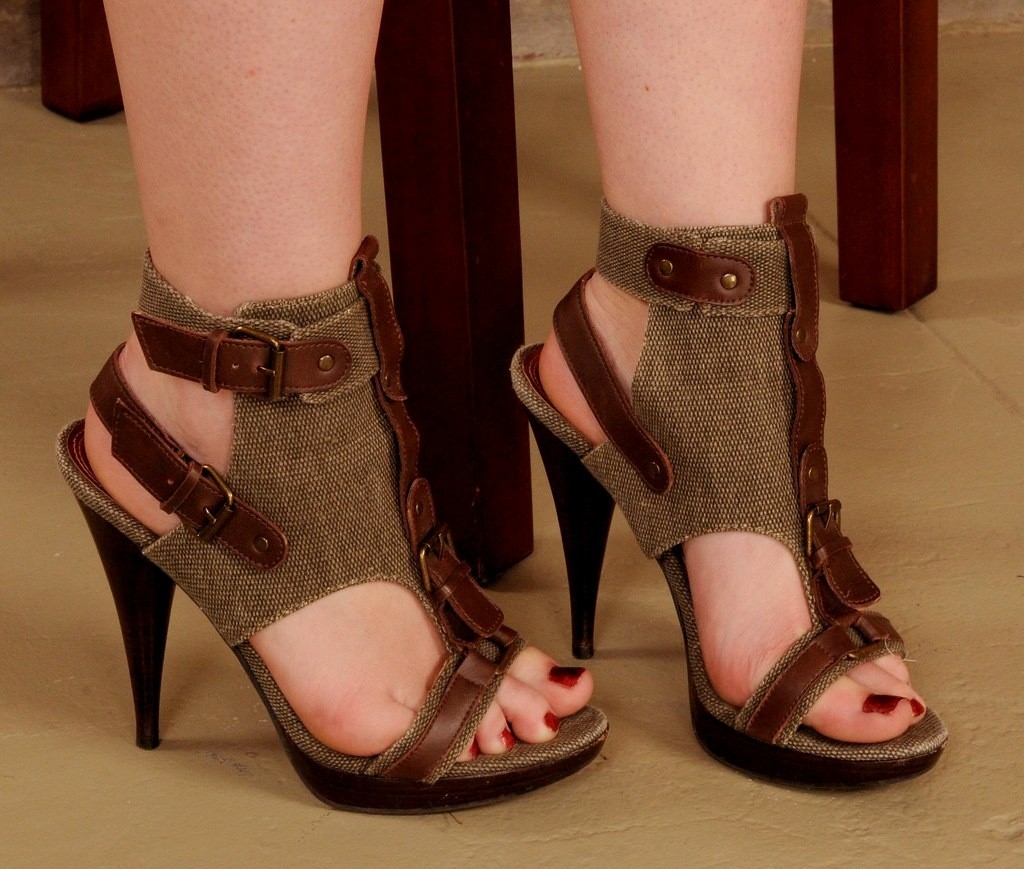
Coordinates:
[55,0,949,817]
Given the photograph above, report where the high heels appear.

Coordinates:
[56,235,609,815]
[510,193,949,792]
